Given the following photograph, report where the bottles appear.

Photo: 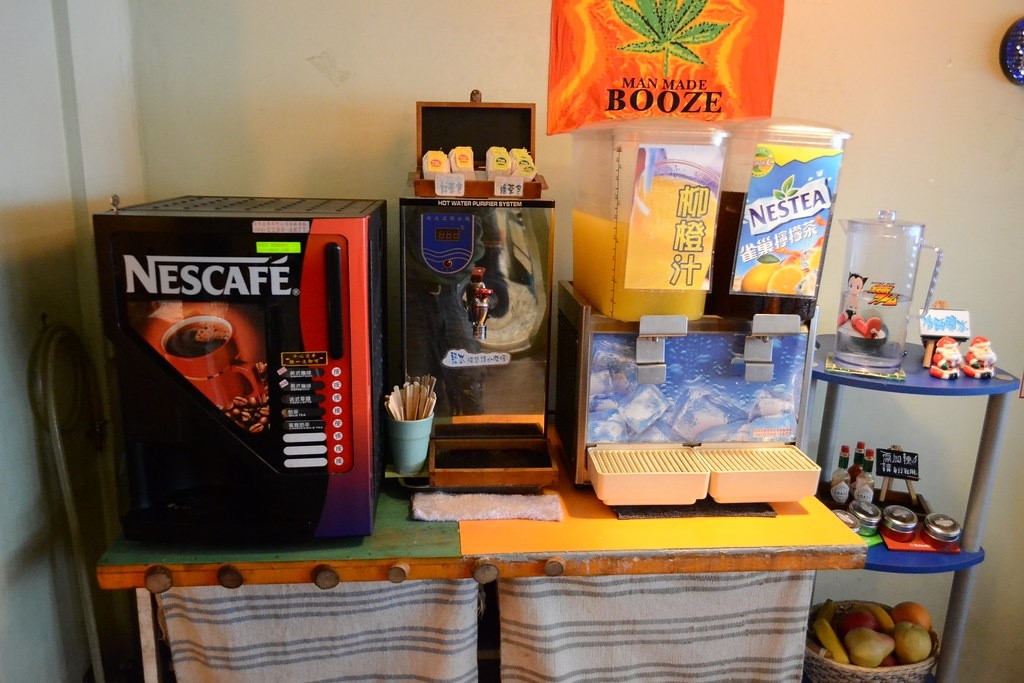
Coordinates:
[834,210,945,374]
[829,439,875,508]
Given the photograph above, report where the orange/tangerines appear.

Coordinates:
[741,247,822,296]
[890,602,931,633]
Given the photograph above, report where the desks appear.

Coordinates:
[95,497,868,683]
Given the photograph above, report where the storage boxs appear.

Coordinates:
[414,100,541,201]
[568,119,728,323]
[710,116,851,324]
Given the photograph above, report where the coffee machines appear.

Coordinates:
[93,195,388,544]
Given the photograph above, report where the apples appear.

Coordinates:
[832,608,878,635]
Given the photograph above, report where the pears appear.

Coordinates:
[891,621,931,665]
[842,627,896,668]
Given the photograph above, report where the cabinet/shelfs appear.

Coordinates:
[801,333,1019,683]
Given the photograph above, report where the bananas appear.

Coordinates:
[811,598,895,666]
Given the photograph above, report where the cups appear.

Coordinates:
[388,413,434,474]
[161,315,262,406]
